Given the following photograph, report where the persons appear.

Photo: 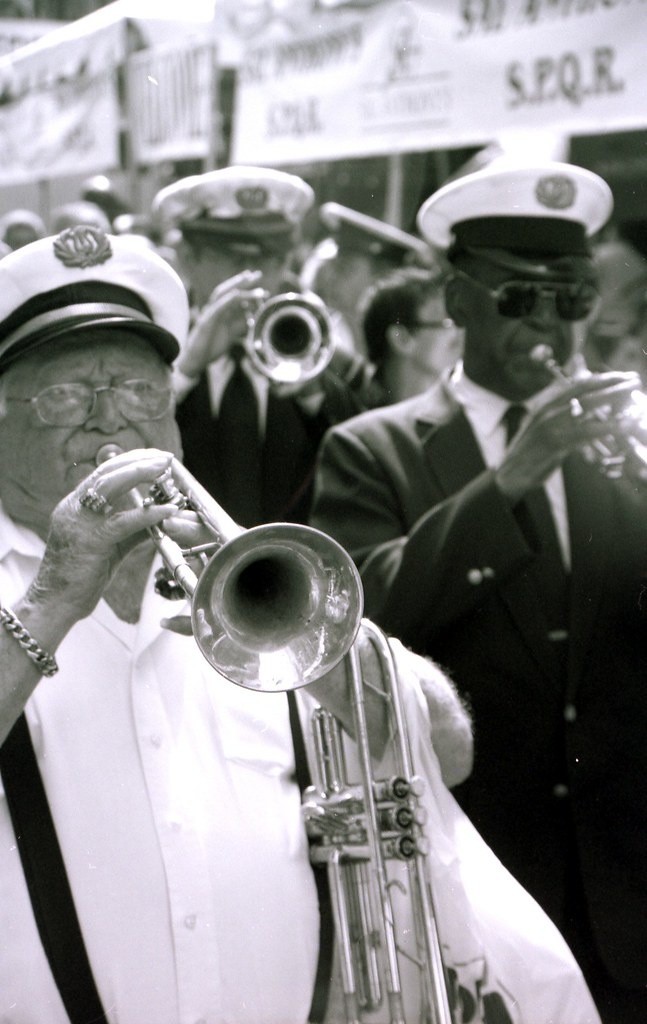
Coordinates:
[0,143,647,1024]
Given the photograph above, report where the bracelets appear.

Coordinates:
[1,607,57,677]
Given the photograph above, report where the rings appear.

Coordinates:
[78,489,112,515]
[569,398,583,418]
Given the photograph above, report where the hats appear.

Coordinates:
[0,224,189,384]
[150,165,316,260]
[416,162,614,275]
[320,201,432,270]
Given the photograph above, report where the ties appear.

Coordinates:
[215,344,264,528]
[506,403,570,670]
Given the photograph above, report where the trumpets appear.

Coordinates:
[237,284,334,385]
[302,615,458,1023]
[532,340,647,491]
[96,444,364,692]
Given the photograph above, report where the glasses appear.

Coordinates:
[4,380,176,427]
[452,268,600,322]
